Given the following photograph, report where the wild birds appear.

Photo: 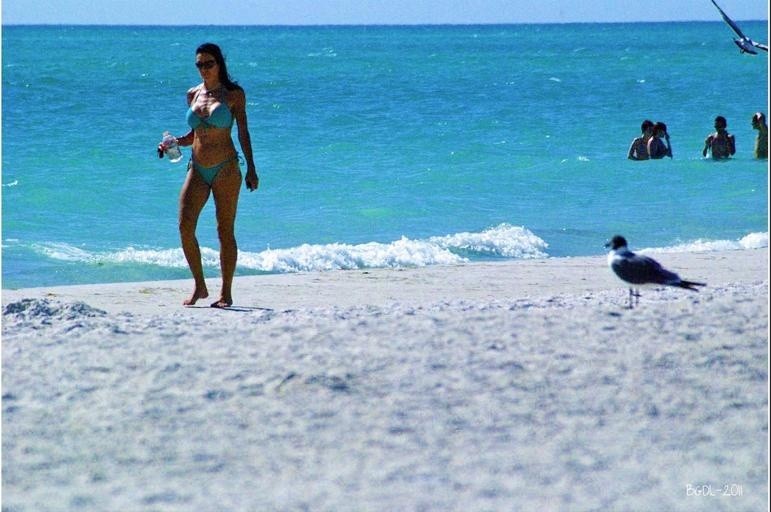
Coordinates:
[606,233,709,311]
[712,0,769,56]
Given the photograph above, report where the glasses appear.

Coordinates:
[195,60,216,69]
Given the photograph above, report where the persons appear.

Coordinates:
[160,43,258,308]
[647,122,673,160]
[752,112,768,160]
[703,116,736,161]
[627,119,653,162]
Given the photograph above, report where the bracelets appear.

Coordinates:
[179,134,187,150]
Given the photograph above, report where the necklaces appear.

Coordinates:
[201,83,221,93]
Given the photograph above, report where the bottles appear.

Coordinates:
[163,132,183,164]
